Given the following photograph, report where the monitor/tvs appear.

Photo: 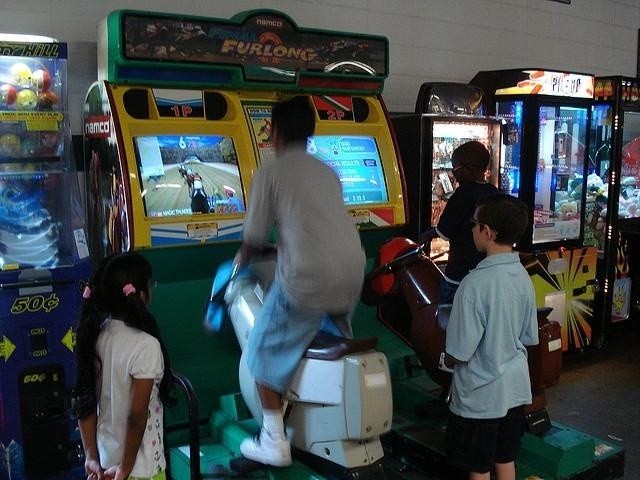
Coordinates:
[134,136,246,217]
[0,175,65,270]
[305,135,389,205]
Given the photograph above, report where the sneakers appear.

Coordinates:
[240,428,292,467]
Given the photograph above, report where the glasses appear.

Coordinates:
[467,215,486,229]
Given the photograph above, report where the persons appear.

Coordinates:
[70,250,178,480]
[435,193,541,479]
[421,140,498,331]
[233,95,366,468]
